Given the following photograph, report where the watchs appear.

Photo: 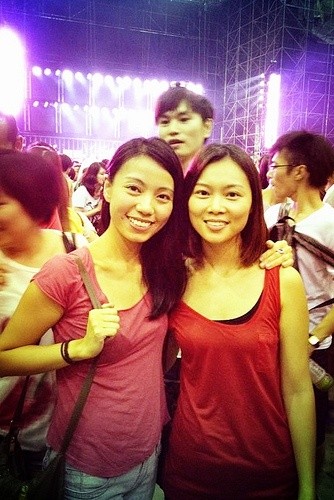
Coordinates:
[308,333,320,348]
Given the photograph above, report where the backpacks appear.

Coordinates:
[265,210,334,310]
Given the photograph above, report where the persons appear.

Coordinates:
[257,129,334,500]
[167,141,316,500]
[155,86,214,177]
[0,109,110,500]
[0,137,294,500]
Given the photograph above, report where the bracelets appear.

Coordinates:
[61,339,79,364]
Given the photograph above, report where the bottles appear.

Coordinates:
[308,359,334,392]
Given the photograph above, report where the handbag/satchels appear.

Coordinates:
[0,434,66,500]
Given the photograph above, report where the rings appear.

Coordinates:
[276,248,284,253]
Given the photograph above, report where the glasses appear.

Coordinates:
[268,163,292,171]
